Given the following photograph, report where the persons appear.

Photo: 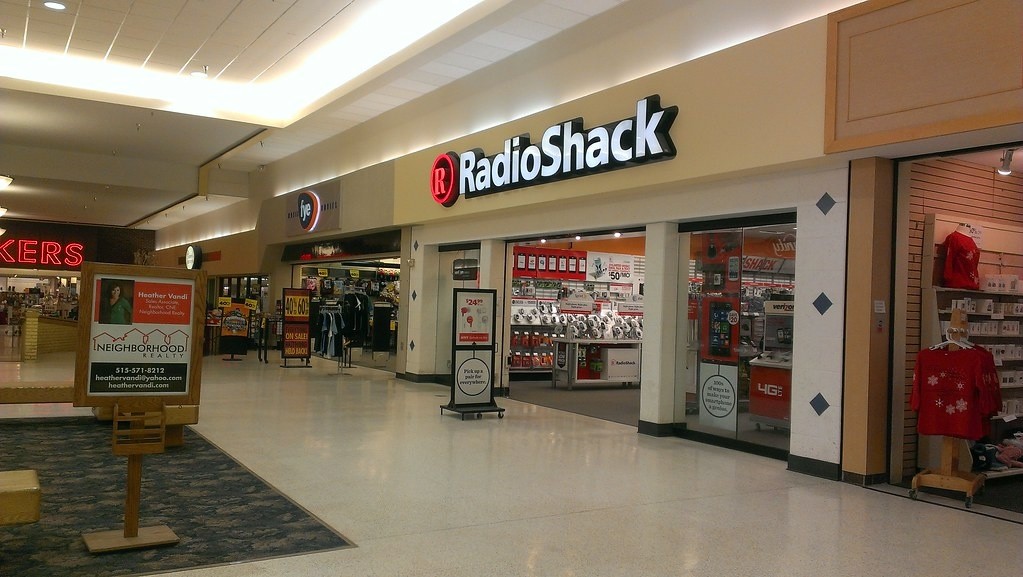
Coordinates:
[101,281,132,325]
[943,222,980,291]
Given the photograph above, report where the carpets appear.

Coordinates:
[0,414,361,577]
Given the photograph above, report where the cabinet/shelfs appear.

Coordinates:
[551,337,642,391]
[916,213,1023,487]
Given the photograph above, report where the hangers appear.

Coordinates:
[346,287,368,294]
[930,328,974,350]
[321,307,339,312]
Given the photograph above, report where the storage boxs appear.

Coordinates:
[940,274,1023,415]
[600,347,639,381]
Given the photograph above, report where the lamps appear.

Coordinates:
[995,148,1017,175]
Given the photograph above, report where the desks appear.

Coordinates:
[748,353,791,432]
